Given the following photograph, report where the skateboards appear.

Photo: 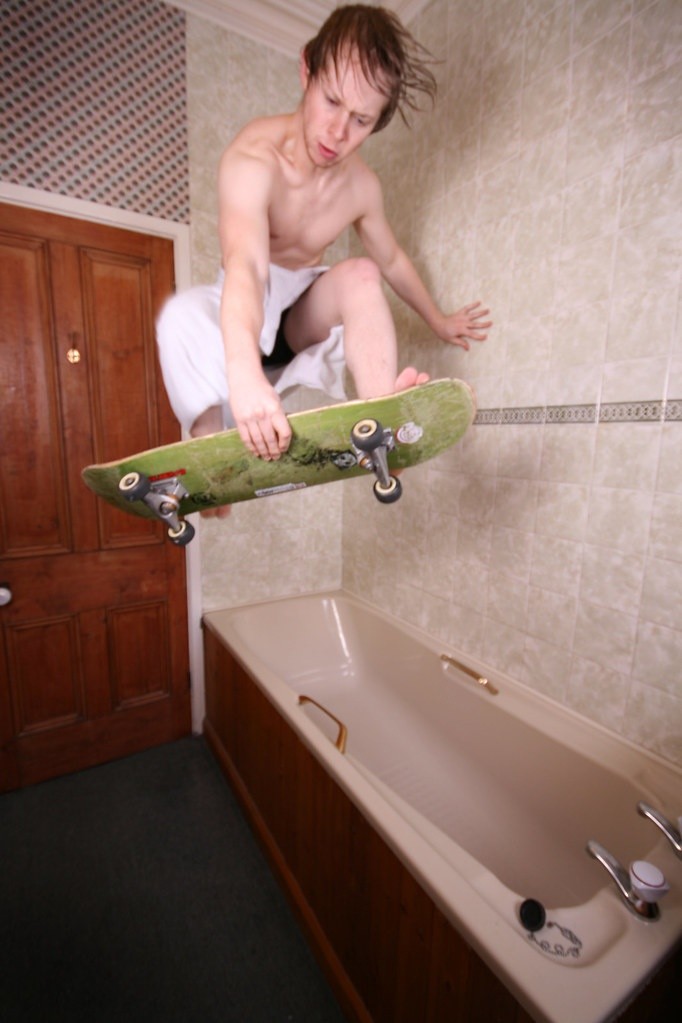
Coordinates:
[79,376,477,546]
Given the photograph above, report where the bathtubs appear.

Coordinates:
[201,586,680,1023]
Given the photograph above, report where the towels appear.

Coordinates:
[157,252,350,442]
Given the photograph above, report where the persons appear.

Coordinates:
[155,4,493,462]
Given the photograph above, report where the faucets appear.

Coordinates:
[585,838,669,923]
[636,799,681,858]
